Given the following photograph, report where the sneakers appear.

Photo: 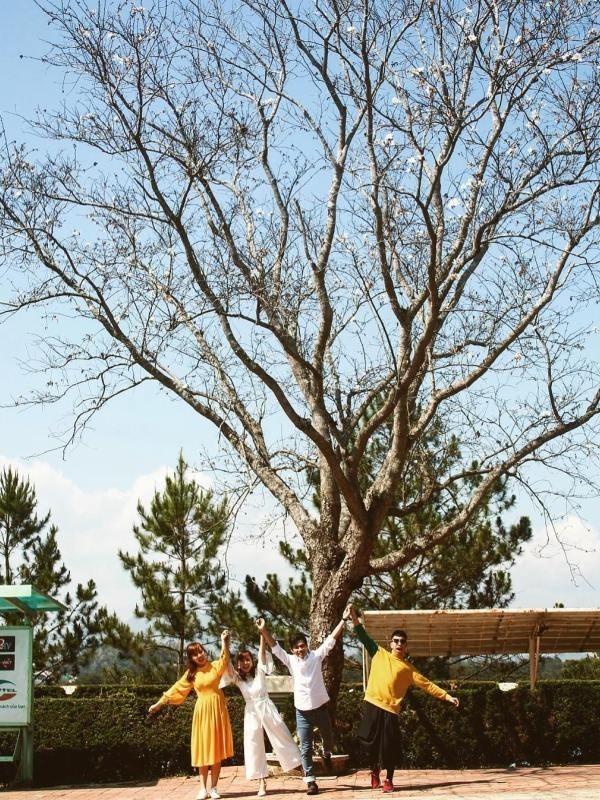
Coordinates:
[383,779,394,792]
[322,750,331,768]
[307,781,318,794]
[368,768,380,789]
[197,790,208,799]
[257,783,268,796]
[208,787,221,798]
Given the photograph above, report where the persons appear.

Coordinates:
[224,619,303,795]
[350,604,460,794]
[148,630,230,800]
[256,603,351,795]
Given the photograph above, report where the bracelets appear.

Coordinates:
[342,617,347,621]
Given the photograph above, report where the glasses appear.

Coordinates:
[392,639,407,644]
